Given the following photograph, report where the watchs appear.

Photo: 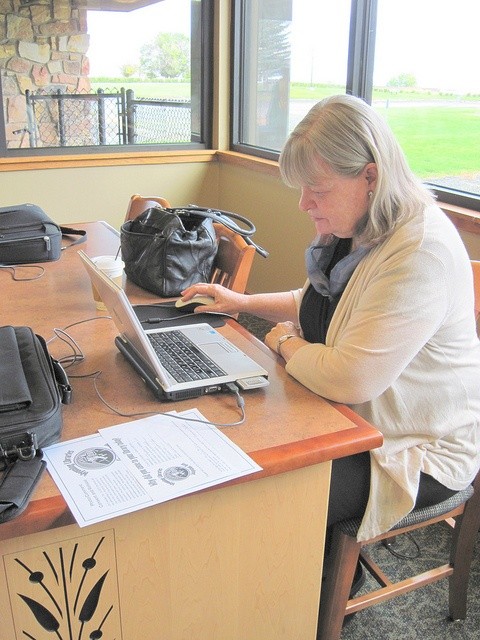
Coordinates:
[279,335,290,343]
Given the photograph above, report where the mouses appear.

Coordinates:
[175,292,240,324]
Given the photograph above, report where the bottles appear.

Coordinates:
[91,255,125,312]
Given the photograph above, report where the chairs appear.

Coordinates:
[124,194,170,222]
[318,258,479,636]
[200,221,255,320]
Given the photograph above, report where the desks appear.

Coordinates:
[0,221,383,636]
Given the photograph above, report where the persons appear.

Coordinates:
[180,94,480,533]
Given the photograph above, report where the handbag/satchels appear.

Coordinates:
[114,208,268,298]
[0,326,71,525]
[0,204,88,264]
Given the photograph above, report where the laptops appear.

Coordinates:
[73,250,272,406]
